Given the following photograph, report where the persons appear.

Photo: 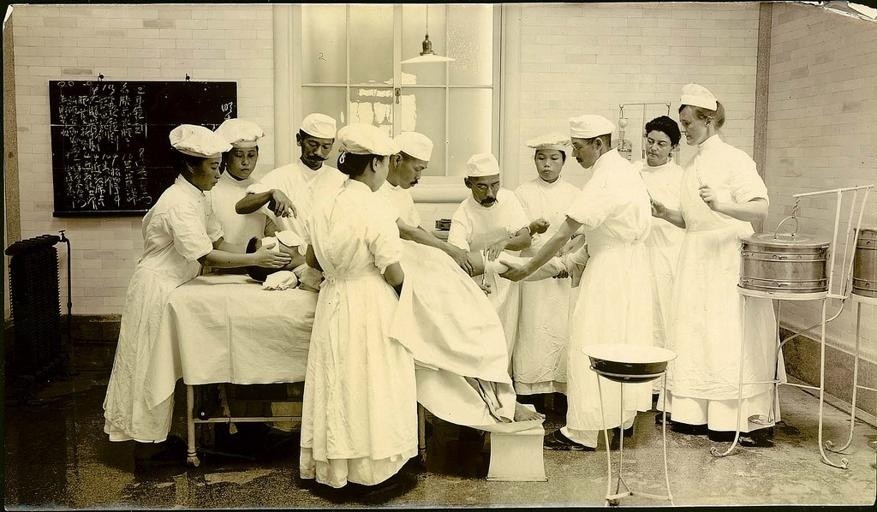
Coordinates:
[235,111,348,257]
[631,115,686,395]
[512,131,582,396]
[297,123,418,488]
[246,231,589,286]
[377,130,474,277]
[647,82,788,447]
[498,115,656,450]
[102,121,292,478]
[445,151,532,260]
[205,117,279,274]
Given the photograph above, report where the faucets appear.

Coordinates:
[57,229,69,242]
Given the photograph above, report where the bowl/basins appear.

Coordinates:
[581,343,679,383]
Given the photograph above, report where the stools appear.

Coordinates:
[480,404,548,486]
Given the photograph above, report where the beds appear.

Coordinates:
[165,265,432,474]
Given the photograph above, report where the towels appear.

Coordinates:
[258,227,308,291]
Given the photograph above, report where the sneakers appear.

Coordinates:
[543,429,592,450]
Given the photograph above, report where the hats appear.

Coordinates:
[681,84,717,111]
[300,113,432,161]
[467,154,500,177]
[169,118,265,158]
[528,116,615,151]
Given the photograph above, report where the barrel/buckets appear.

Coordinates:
[852,226,877,300]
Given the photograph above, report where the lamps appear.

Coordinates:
[401,6,456,68]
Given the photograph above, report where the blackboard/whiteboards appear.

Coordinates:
[48,79,238,217]
